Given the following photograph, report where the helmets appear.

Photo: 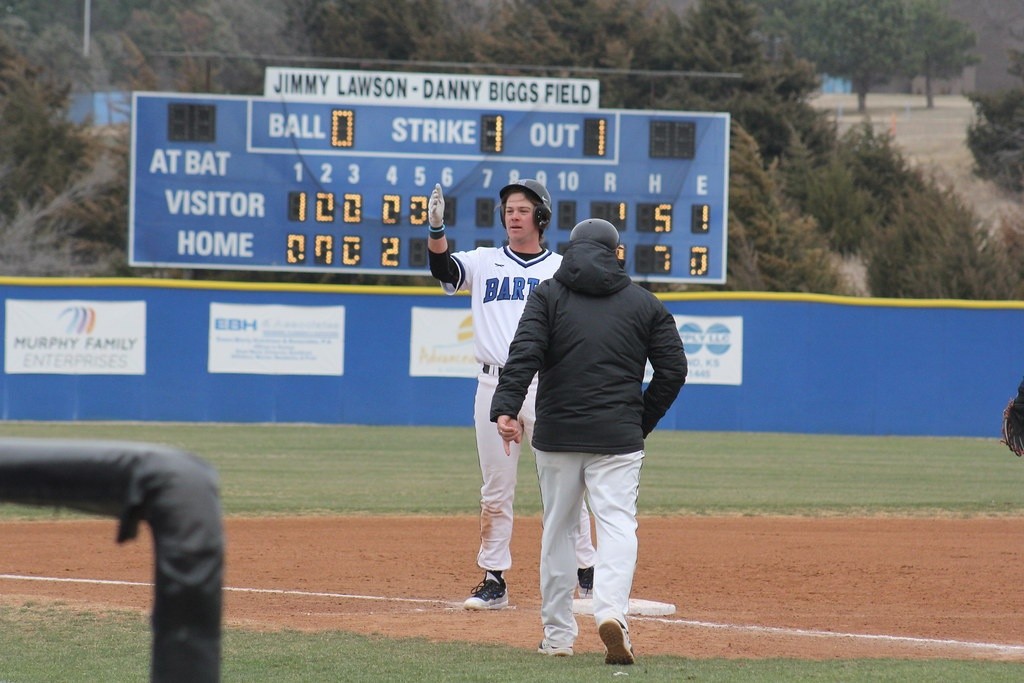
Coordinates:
[499,178,551,228]
[569,218,619,252]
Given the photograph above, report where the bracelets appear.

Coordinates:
[429,224,445,240]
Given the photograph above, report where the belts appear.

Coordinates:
[483,364,503,377]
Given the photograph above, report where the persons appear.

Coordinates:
[428,179,596,610]
[490,218,688,663]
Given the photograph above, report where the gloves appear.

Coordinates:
[428,183,446,231]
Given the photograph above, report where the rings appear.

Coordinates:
[499,430,503,434]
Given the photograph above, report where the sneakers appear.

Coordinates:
[578,567,595,598]
[598,618,634,665]
[463,571,508,611]
[536,639,573,658]
